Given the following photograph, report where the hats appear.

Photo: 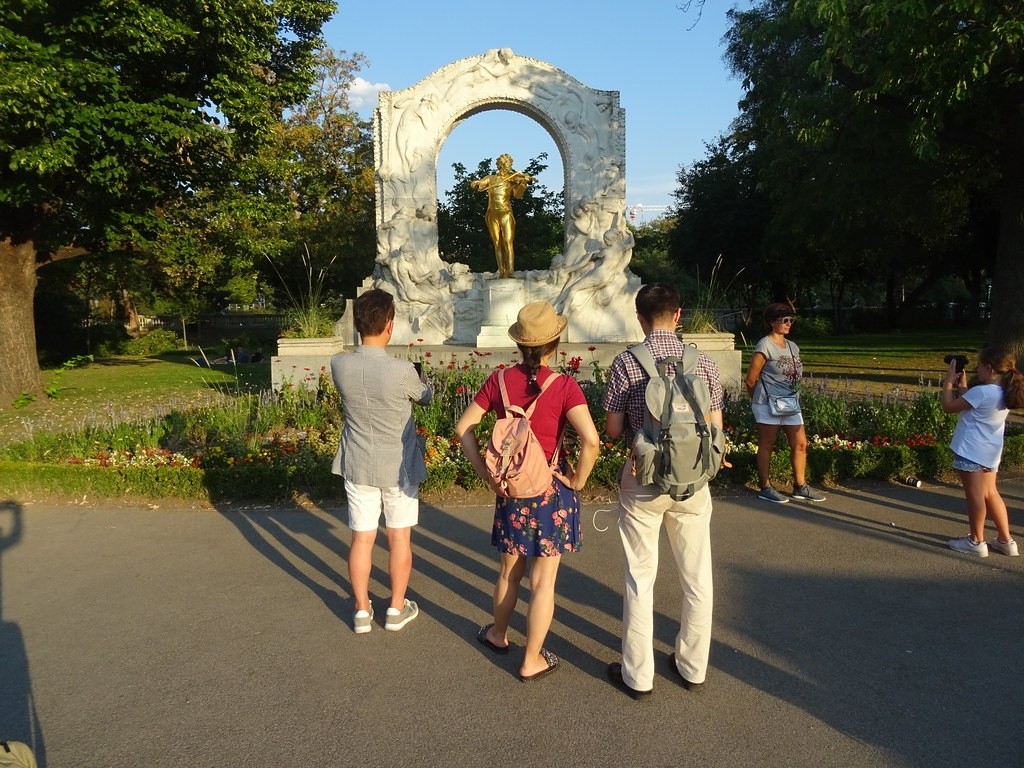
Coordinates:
[508,300,570,346]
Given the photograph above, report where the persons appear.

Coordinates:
[470,154,532,278]
[330,289,432,633]
[941,347,1024,558]
[455,300,599,685]
[746,303,826,504]
[603,282,726,698]
[209,346,265,365]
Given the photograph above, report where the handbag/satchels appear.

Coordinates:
[767,393,800,416]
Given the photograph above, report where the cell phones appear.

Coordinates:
[413,363,421,378]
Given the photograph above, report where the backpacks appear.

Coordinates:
[624,341,727,502]
[484,368,564,498]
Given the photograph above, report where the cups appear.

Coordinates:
[906,476,921,488]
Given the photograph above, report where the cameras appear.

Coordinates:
[944,355,969,374]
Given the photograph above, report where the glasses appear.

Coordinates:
[776,317,794,324]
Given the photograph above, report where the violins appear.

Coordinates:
[503,170,540,184]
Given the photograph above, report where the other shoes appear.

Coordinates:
[667,649,705,691]
[608,662,653,699]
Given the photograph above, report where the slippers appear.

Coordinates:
[475,622,511,653]
[517,649,559,683]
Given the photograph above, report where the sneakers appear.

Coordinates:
[757,484,790,503]
[991,536,1020,556]
[948,534,990,558]
[385,598,418,631]
[352,598,375,633]
[791,483,826,502]
